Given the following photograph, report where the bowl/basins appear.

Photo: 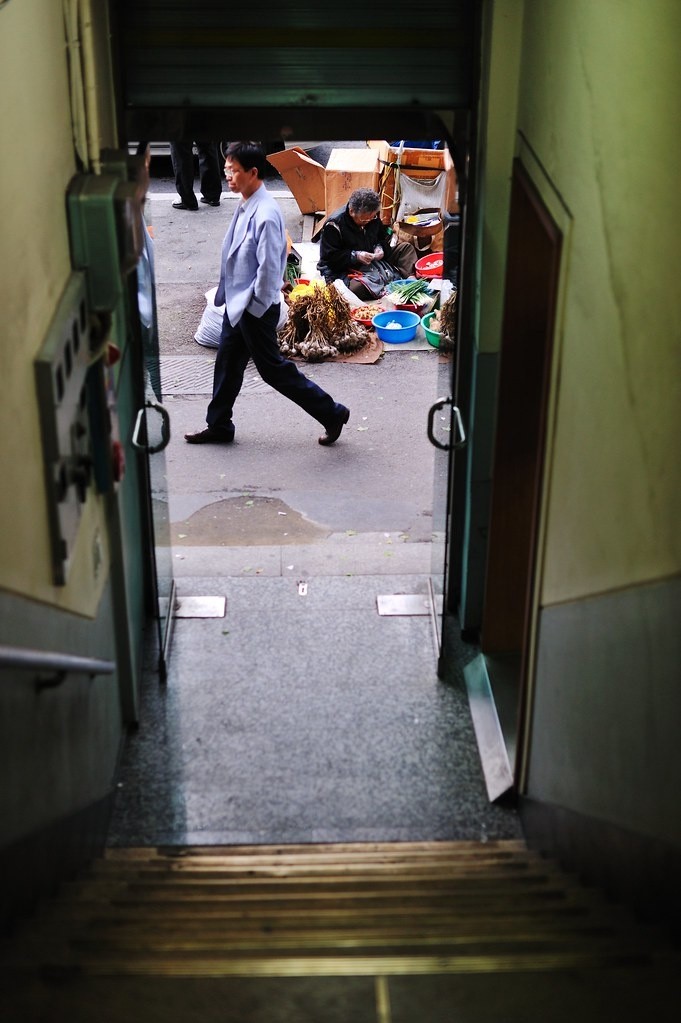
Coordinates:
[350,306,386,326]
[387,279,434,295]
[371,310,420,343]
[415,253,444,274]
[416,270,443,279]
[420,312,442,348]
[394,303,426,314]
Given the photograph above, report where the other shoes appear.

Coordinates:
[184,422,235,444]
[172,201,199,211]
[200,196,220,207]
[318,406,350,446]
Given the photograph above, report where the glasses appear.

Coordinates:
[224,169,249,176]
[352,212,378,223]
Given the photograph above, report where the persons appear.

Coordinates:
[317,187,418,301]
[170,142,222,210]
[185,141,350,446]
